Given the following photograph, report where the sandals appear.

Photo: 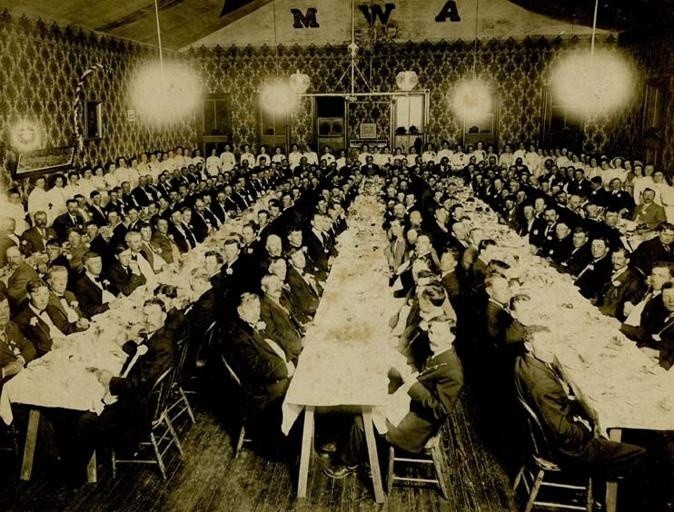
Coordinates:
[324,465,360,479]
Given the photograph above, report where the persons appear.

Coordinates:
[0,142,674,511]
